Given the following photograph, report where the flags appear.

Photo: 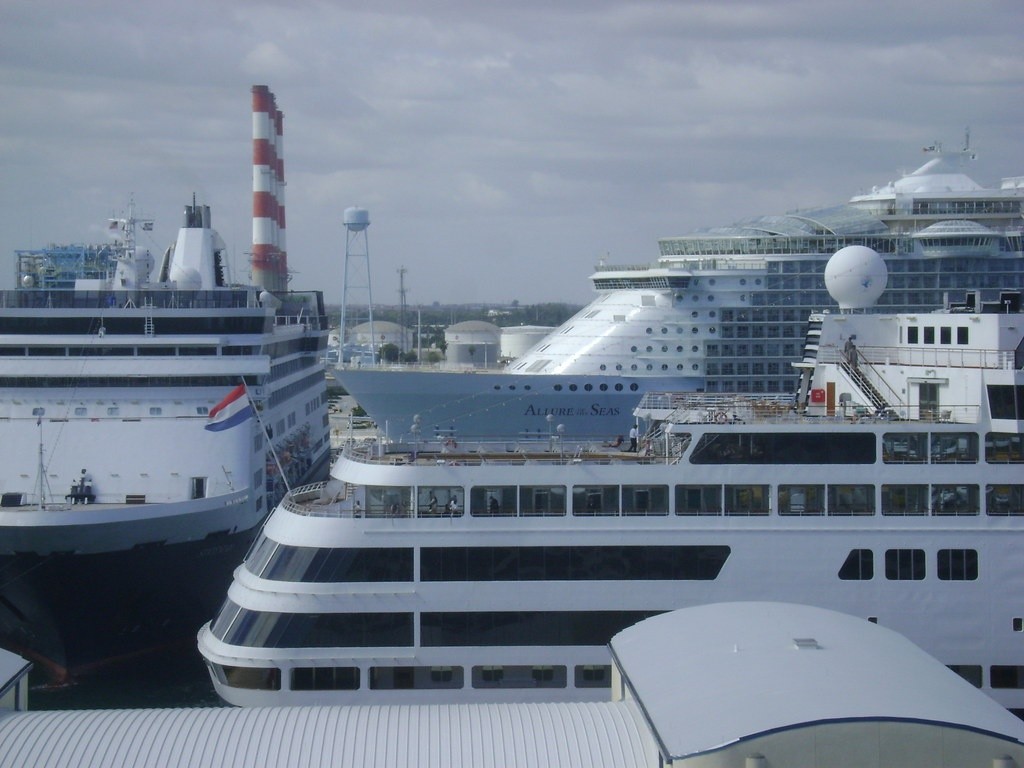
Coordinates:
[205,383,255,432]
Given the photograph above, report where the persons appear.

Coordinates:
[489,496,500,515]
[627,424,641,452]
[390,502,400,515]
[844,335,859,373]
[450,499,460,513]
[427,494,439,514]
[354,499,362,518]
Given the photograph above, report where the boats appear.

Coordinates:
[197,246,1023,723]
[0,86,330,686]
[327,126,1024,436]
